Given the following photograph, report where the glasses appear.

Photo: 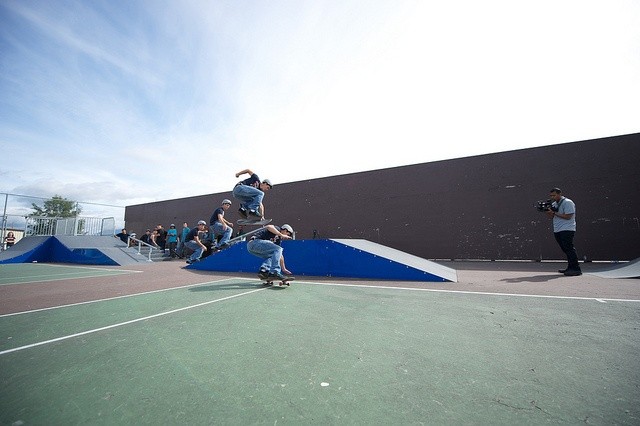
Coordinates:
[146,230,150,232]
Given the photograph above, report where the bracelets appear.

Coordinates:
[552,212,555,216]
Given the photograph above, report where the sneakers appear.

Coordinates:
[186,259,192,264]
[221,243,229,248]
[213,249,221,253]
[559,268,567,273]
[269,272,286,280]
[238,208,248,218]
[258,270,269,280]
[249,210,263,217]
[565,270,581,276]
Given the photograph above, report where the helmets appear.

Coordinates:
[222,199,232,205]
[262,179,273,189]
[280,224,293,234]
[197,220,206,225]
[130,233,137,237]
[171,224,175,226]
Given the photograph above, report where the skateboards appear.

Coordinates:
[171,249,183,259]
[261,277,295,286]
[237,219,272,226]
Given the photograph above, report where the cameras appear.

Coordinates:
[536,200,556,211]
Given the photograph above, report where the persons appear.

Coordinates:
[232,169,273,221]
[140,229,153,246]
[165,224,179,248]
[151,228,160,241]
[156,224,168,250]
[246,224,293,279]
[4,232,16,248]
[235,225,245,238]
[545,188,582,276]
[184,220,207,265]
[179,222,191,259]
[208,199,234,250]
[114,229,130,243]
